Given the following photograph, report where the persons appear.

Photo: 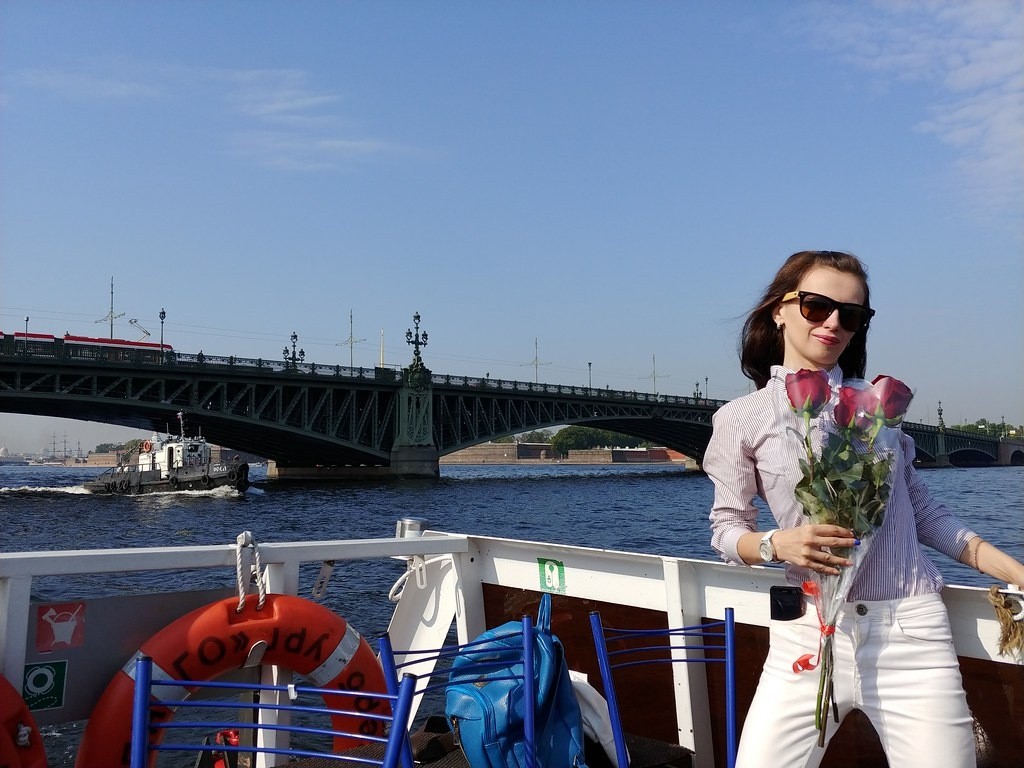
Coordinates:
[703,251,1024,768]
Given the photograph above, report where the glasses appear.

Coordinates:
[782,291,875,332]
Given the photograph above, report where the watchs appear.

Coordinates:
[759,529,782,562]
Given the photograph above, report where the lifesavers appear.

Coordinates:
[201,475,210,485]
[169,476,178,486]
[76,591,393,766]
[144,441,152,452]
[228,463,250,491]
[104,479,142,494]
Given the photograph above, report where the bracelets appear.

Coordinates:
[974,541,985,574]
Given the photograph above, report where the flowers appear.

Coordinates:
[781,368,915,748]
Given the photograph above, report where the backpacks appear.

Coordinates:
[445,593,588,768]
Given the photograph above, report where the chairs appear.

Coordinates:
[375,613,536,768]
[588,607,734,768]
[129,655,418,768]
